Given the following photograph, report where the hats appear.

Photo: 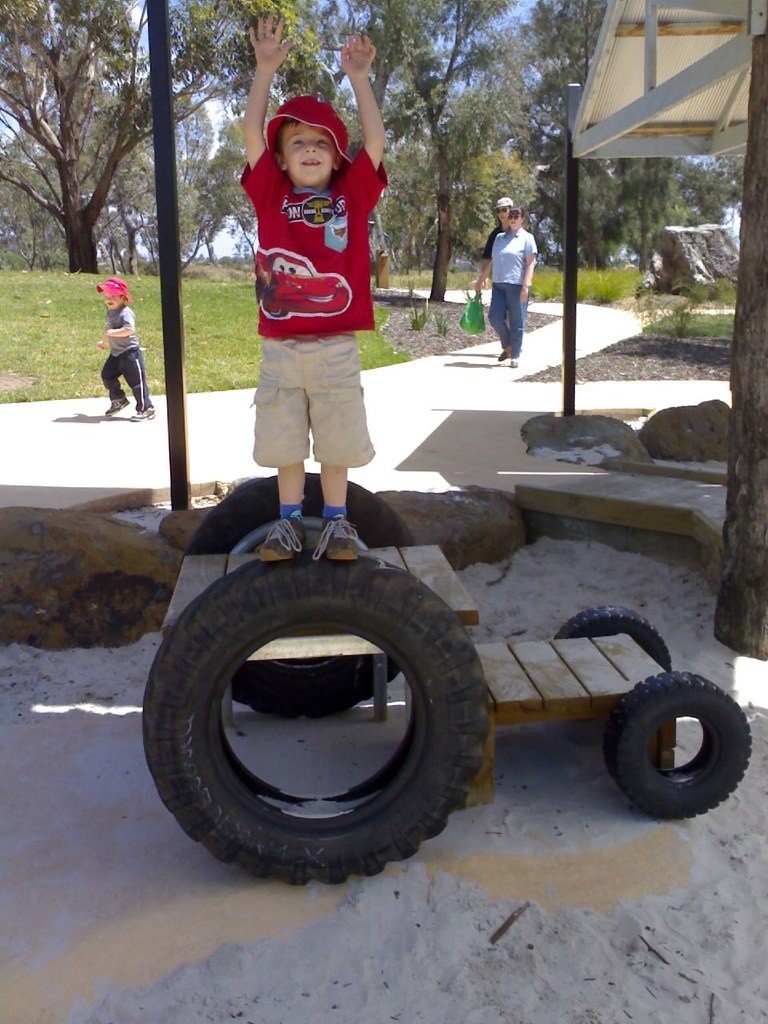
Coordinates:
[267,94,354,166]
[97,277,131,304]
[494,197,513,209]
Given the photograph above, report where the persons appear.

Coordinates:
[477,198,537,366]
[242,12,385,562]
[96,276,157,421]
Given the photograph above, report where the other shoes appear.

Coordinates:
[511,360,518,368]
[498,351,512,361]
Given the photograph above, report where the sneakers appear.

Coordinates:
[131,408,155,422]
[312,514,359,559]
[106,398,130,416]
[259,517,305,562]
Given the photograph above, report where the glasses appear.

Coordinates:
[496,208,509,213]
[508,216,521,220]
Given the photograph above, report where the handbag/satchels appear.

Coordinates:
[460,293,485,335]
